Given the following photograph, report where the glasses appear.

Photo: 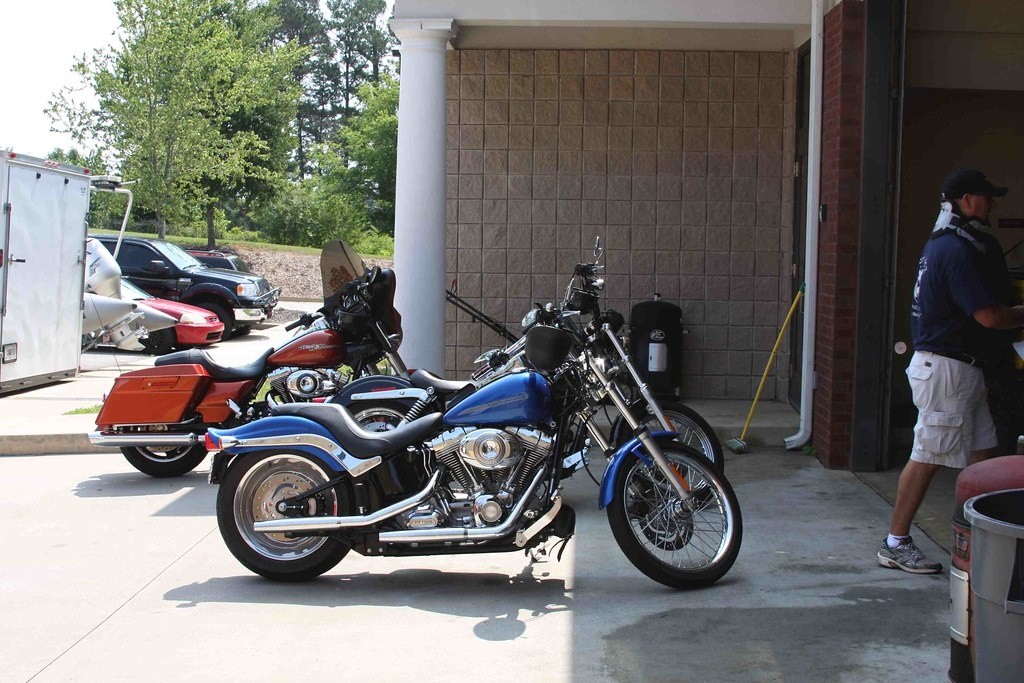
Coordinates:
[972,192,994,202]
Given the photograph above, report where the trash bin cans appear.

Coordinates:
[965,489,1024,683]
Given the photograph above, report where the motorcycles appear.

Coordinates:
[199,231,745,591]
[83,238,423,480]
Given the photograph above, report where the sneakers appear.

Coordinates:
[877,538,943,573]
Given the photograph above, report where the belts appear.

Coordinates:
[933,351,987,369]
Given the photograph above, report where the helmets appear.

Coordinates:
[565,286,599,315]
[340,306,383,334]
[524,325,571,370]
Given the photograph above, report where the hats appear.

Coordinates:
[941,168,1007,200]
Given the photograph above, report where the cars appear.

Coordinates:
[81,277,225,356]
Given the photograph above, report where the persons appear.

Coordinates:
[876,167,1024,575]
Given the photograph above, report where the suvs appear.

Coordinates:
[186,249,251,274]
[83,232,283,341]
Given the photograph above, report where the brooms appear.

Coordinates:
[723,282,806,455]
